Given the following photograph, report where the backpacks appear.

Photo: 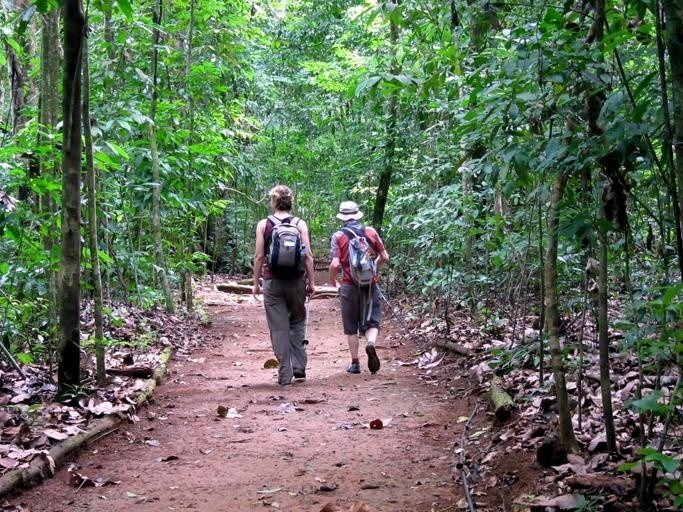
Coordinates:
[348,228,379,286]
[266,217,306,280]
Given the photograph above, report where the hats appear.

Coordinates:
[337,202,363,221]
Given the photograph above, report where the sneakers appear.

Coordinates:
[293,368,306,381]
[366,344,380,372]
[279,378,291,385]
[347,363,360,373]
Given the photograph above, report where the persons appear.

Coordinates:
[249,185,315,385]
[327,197,388,375]
